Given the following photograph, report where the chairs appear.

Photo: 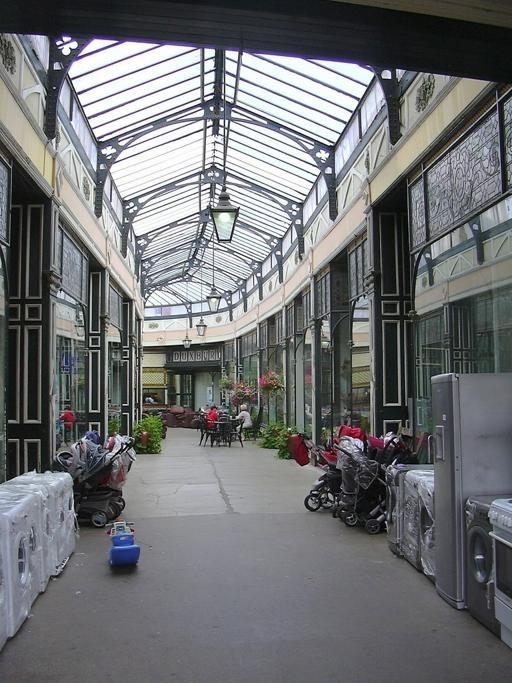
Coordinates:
[196,411,257,449]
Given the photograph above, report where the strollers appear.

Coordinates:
[299,425,415,534]
[52,429,137,528]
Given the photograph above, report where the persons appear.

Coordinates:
[206,406,218,429]
[236,404,253,441]
[144,394,158,405]
[60,405,77,428]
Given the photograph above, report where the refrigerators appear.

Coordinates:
[426,370,512,613]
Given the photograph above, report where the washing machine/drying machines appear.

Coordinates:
[381,464,436,585]
[460,493,512,640]
[0,470,79,654]
[489,494,512,648]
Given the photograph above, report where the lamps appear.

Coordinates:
[204,48,244,246]
[181,280,193,351]
[203,231,226,315]
[193,265,210,338]
